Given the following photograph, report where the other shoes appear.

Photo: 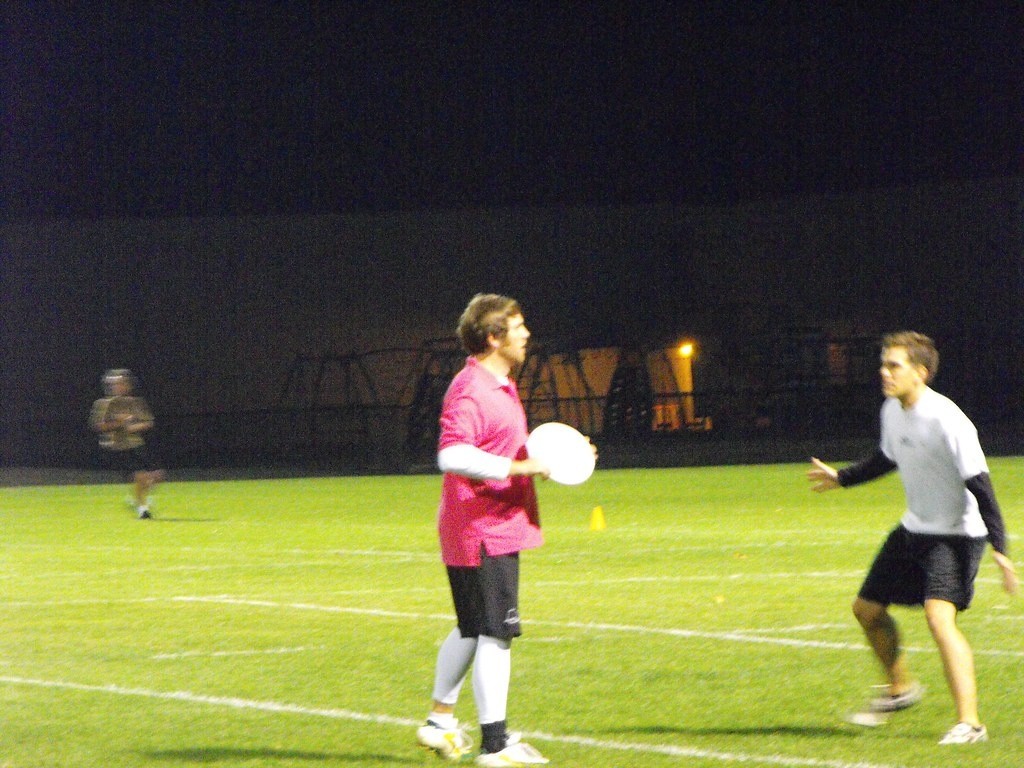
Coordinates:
[136,504,150,518]
[936,722,989,744]
[872,685,925,711]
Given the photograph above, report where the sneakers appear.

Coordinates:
[414,712,472,762]
[474,731,550,767]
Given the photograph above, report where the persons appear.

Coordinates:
[88,369,163,519]
[419,295,599,765]
[807,331,1018,744]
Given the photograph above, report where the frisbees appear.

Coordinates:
[523,420,600,488]
